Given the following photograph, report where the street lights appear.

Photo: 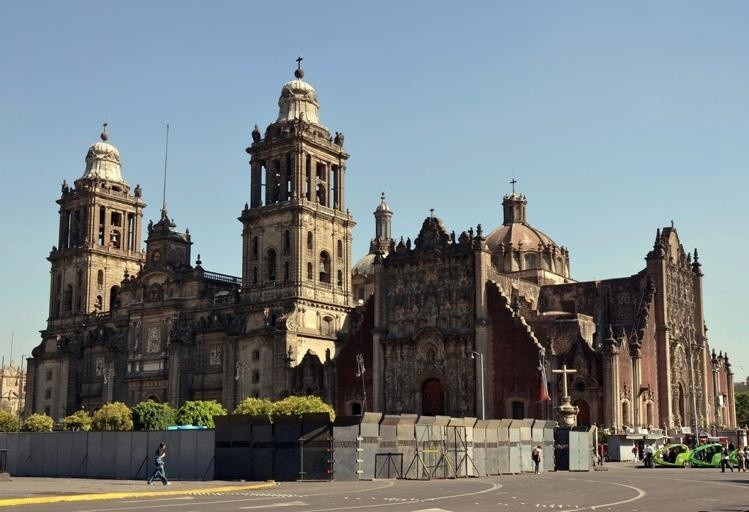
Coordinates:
[234,360,249,405]
[8,366,24,420]
[710,348,724,424]
[355,353,368,411]
[470,350,485,419]
[102,368,115,404]
[724,352,734,426]
[718,349,724,393]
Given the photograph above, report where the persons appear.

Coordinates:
[598,440,749,473]
[146,442,171,488]
[531,445,543,475]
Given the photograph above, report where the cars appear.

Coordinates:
[52,415,65,431]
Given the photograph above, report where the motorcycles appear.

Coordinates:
[652,443,691,468]
[729,446,749,468]
[687,443,724,468]
